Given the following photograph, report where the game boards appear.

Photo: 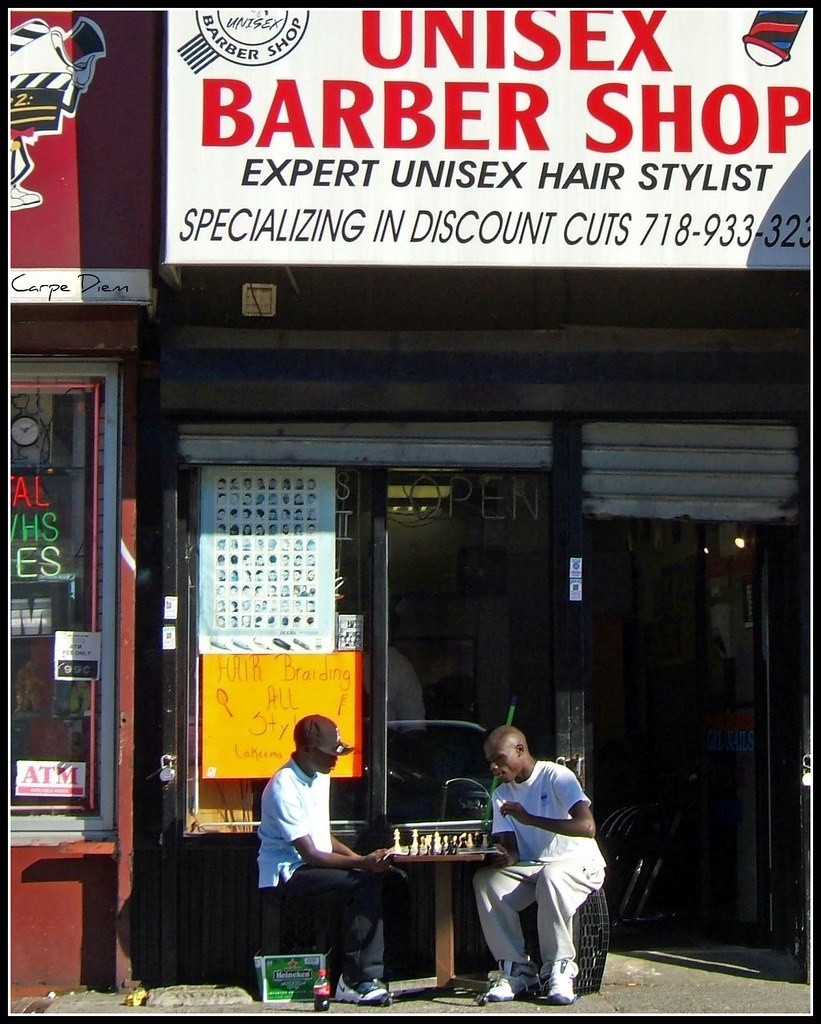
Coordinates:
[383,843,504,860]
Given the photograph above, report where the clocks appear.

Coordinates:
[11,415,40,447]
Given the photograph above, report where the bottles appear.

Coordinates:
[392,827,489,856]
[313,969,330,1011]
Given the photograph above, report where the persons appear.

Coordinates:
[215,476,318,629]
[472,726,608,1006]
[364,629,427,763]
[256,714,407,1005]
[339,620,362,647]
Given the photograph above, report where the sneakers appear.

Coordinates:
[334,973,393,1006]
[486,960,541,1002]
[542,959,578,1004]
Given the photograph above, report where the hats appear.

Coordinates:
[294,714,356,756]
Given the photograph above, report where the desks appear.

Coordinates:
[388,854,491,995]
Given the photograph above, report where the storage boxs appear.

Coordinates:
[253,944,334,1003]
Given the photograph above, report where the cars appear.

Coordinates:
[327,720,493,820]
